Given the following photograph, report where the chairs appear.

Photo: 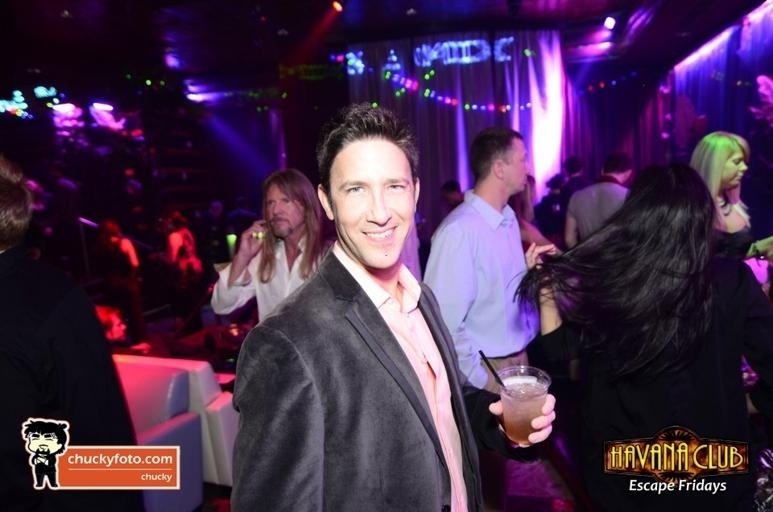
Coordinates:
[112,353,245,488]
[113,361,204,512]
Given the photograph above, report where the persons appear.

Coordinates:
[685,129,773,264]
[17,155,260,378]
[433,147,638,261]
[207,166,335,328]
[505,161,773,511]
[415,123,540,510]
[224,97,558,510]
[0,150,151,512]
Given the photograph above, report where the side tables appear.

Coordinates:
[169,322,259,367]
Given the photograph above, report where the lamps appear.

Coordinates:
[333,0,348,11]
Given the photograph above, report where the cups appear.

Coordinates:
[494,365,551,444]
